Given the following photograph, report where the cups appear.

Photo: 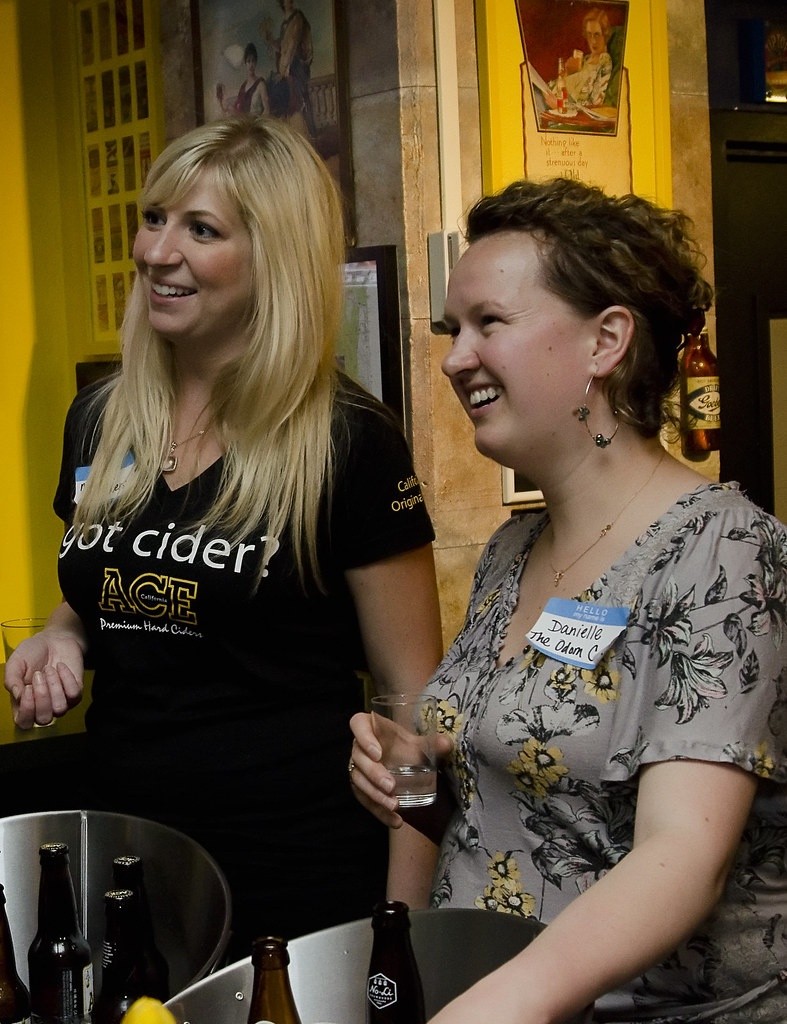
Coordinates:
[371,694,438,808]
[1,618,58,728]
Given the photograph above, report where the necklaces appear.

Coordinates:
[157,425,209,473]
[547,448,666,587]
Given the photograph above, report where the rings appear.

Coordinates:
[348,760,357,783]
[34,716,56,729]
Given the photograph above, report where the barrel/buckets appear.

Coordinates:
[0,811,233,997]
[164,909,594,1024]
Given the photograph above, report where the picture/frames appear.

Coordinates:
[337,243,403,431]
[514,0,630,139]
[189,0,356,254]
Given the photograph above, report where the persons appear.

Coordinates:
[1,118,445,952]
[345,181,787,1024]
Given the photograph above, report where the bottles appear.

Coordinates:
[366,901,427,1024]
[247,934,302,1023]
[0,884,29,1023]
[113,855,169,1003]
[28,843,96,1024]
[98,888,152,1023]
[684,325,720,454]
